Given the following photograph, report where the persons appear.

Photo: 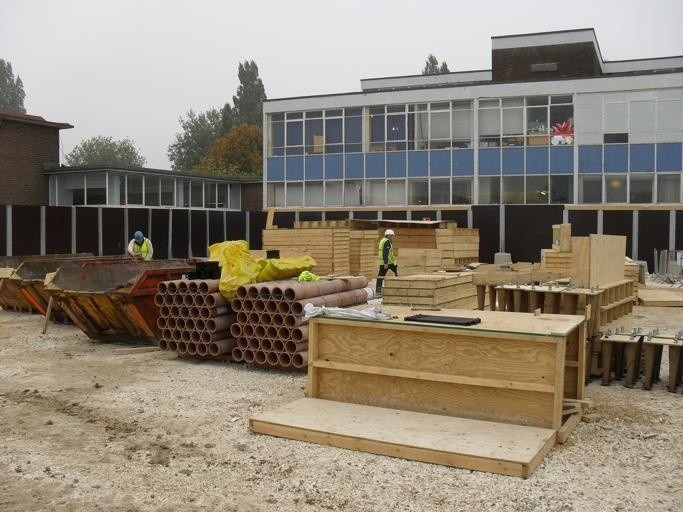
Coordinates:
[127,231,153,261]
[374,229,397,298]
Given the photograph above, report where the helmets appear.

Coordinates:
[385,229,395,236]
[134,230,143,241]
